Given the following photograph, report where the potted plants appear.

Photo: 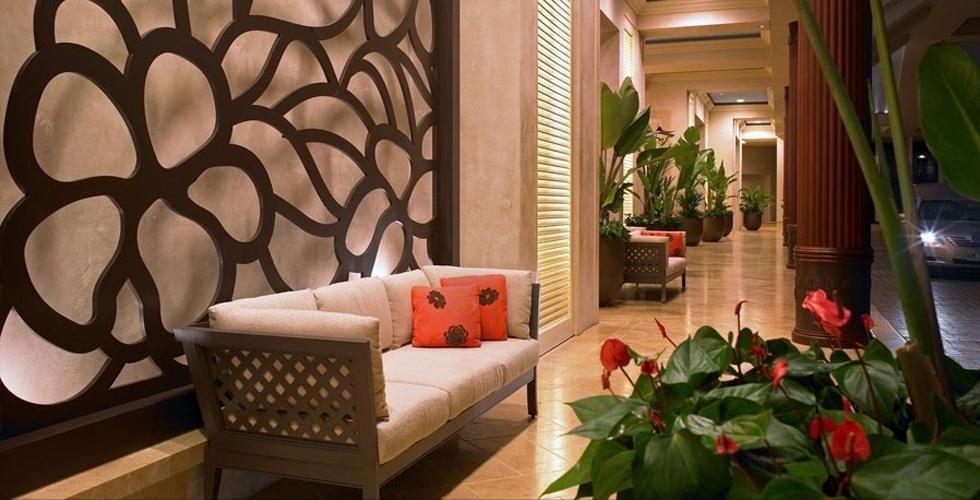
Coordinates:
[602,78,694,306]
[626,77,778,246]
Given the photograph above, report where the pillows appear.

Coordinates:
[419,264,532,339]
[378,269,430,349]
[411,285,481,348]
[209,288,319,329]
[217,307,390,418]
[312,277,393,351]
[440,274,508,342]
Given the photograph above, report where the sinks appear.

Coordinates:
[171,264,545,500]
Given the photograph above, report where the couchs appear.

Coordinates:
[171,264,545,500]
[621,231,687,302]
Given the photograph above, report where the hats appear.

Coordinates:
[899,196,980,268]
